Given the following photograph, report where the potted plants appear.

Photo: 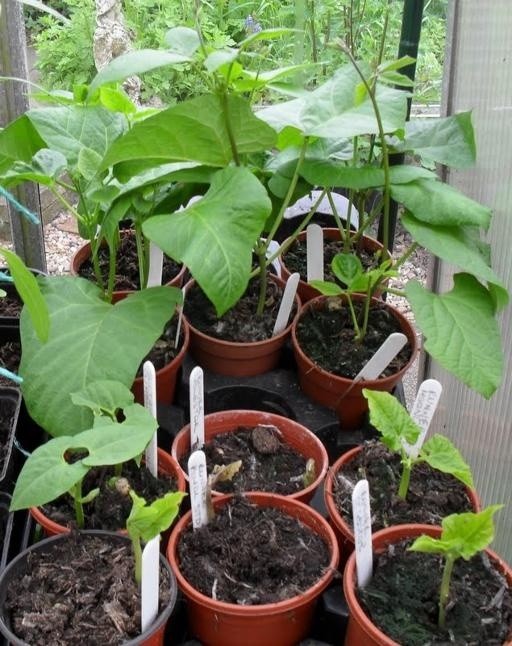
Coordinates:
[1,381,184,645]
[324,388,511,645]
[0,0,509,444]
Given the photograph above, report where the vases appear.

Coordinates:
[167,491,340,645]
[171,409,340,505]
